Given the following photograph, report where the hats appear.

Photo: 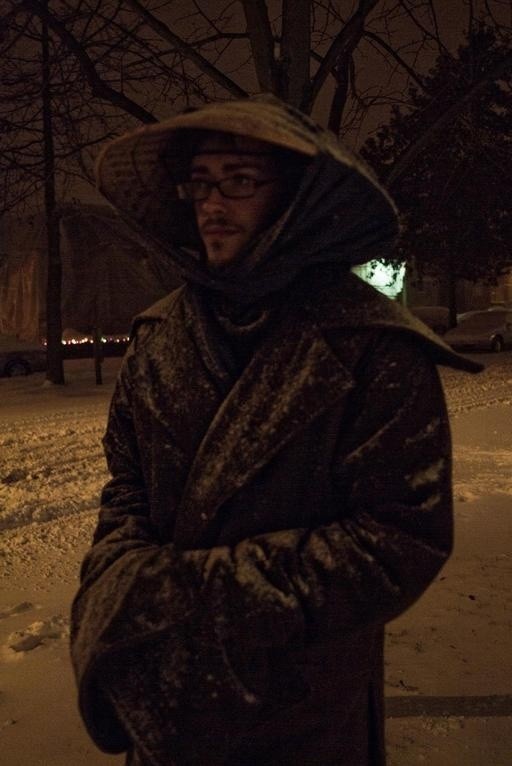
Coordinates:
[94,92,398,266]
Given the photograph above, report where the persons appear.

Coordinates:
[62,90,485,766]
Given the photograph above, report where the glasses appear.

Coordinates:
[175,175,279,201]
